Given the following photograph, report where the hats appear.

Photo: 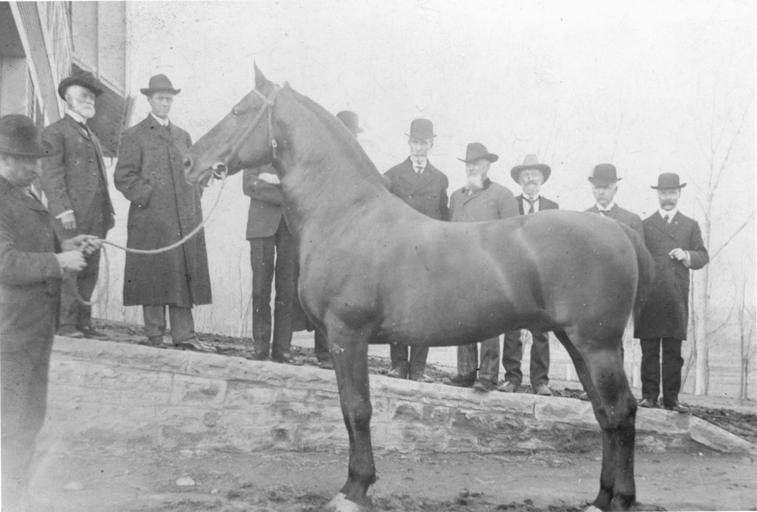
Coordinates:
[139,72,183,97]
[57,74,106,98]
[587,163,624,186]
[0,113,59,158]
[650,171,688,191]
[458,142,500,165]
[337,111,364,135]
[404,117,439,142]
[508,152,553,186]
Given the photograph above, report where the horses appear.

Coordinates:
[182,61,656,512]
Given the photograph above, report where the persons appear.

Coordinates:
[243,162,303,366]
[382,119,448,382]
[0,115,105,508]
[449,143,519,392]
[294,111,365,369]
[497,155,559,395]
[113,74,217,351]
[40,70,116,340]
[634,173,709,413]
[579,164,645,401]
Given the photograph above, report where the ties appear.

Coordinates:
[521,197,540,214]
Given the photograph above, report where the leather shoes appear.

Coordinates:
[442,375,520,392]
[62,321,110,342]
[175,335,219,354]
[384,368,435,385]
[245,347,301,368]
[148,336,166,350]
[533,383,553,397]
[637,397,689,411]
